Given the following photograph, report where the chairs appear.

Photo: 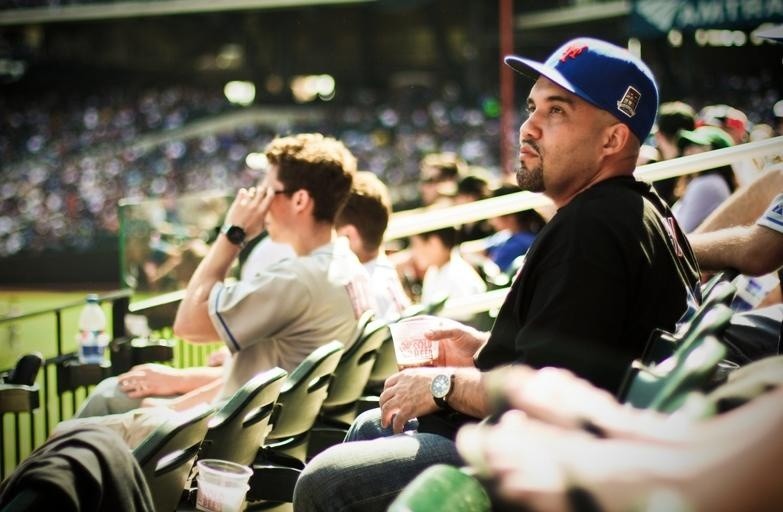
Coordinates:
[653,303,741,356]
[267,340,356,512]
[693,270,730,298]
[184,367,300,512]
[127,406,215,511]
[393,459,500,511]
[312,313,398,460]
[367,298,449,396]
[670,282,744,338]
[621,334,732,418]
[666,388,724,427]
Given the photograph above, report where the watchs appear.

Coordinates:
[429,370,455,410]
[220,223,248,250]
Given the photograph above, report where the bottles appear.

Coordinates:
[79,294,106,365]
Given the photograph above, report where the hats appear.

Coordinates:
[502,36,660,144]
[674,126,735,150]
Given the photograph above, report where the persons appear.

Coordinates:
[69,173,411,416]
[455,175,495,238]
[45,132,360,448]
[206,155,294,364]
[409,227,486,307]
[479,365,783,512]
[478,185,545,285]
[419,153,459,204]
[293,39,704,512]
[653,101,692,160]
[685,161,782,361]
[667,125,737,235]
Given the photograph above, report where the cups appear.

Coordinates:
[197,458,254,486]
[388,319,436,371]
[196,478,250,512]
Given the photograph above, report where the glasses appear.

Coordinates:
[264,188,298,196]
